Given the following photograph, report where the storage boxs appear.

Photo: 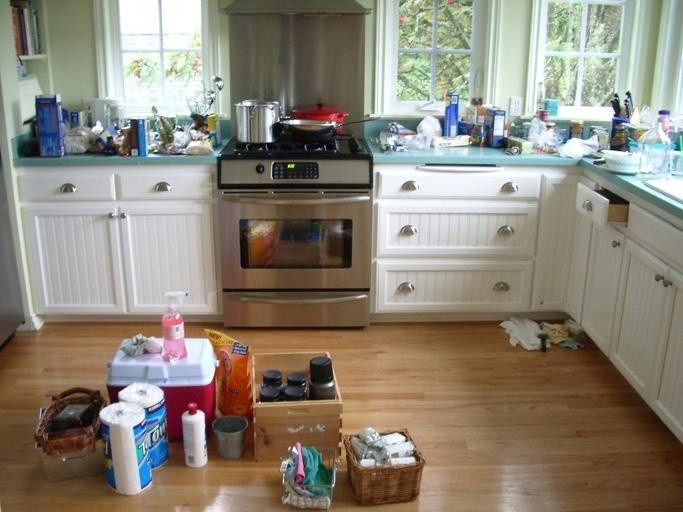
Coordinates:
[248,350,343,462]
[103,337,219,444]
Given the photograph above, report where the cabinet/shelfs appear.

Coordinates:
[564,154,633,373]
[609,181,683,447]
[372,137,583,324]
[16,154,224,329]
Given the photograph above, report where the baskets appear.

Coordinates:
[34,387,106,456]
[342,427,427,506]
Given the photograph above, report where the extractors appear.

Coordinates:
[224,0,372,15]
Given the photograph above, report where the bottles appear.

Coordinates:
[182,403,207,468]
[568,120,584,139]
[636,122,671,178]
[308,356,335,400]
[657,110,670,136]
[610,117,627,151]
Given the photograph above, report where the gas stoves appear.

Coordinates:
[217,138,373,190]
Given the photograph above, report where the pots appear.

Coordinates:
[288,103,350,135]
[234,98,281,143]
[280,116,382,143]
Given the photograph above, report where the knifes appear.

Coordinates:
[610,90,640,123]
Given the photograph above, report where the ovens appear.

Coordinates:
[216,189,373,327]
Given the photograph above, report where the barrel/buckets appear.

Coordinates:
[211,414,249,459]
